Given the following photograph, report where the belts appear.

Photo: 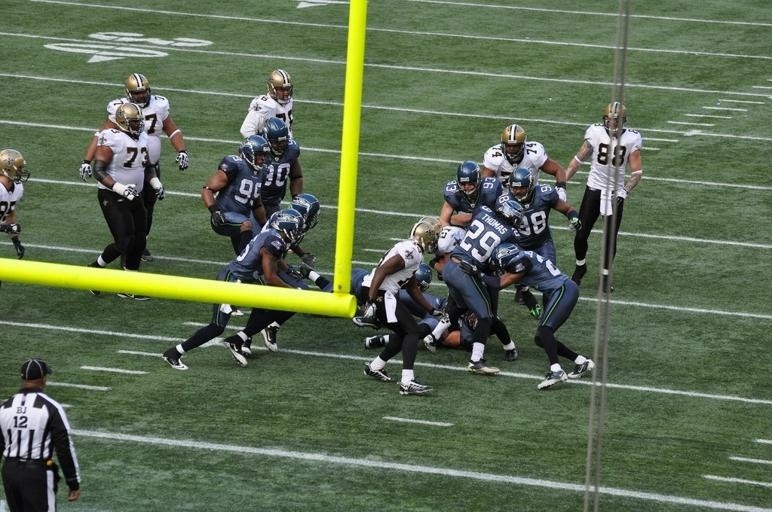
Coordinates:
[6,457,44,464]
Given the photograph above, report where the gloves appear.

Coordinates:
[13,237,24,258]
[287,263,303,282]
[2,223,21,238]
[300,252,317,267]
[150,177,164,200]
[568,217,582,232]
[80,160,92,182]
[112,182,139,201]
[364,301,377,319]
[555,187,566,202]
[208,205,225,226]
[176,150,189,170]
[432,310,444,318]
[459,260,479,277]
[612,189,628,206]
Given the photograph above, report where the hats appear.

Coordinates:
[22,358,52,379]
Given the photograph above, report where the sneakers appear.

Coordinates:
[505,348,518,361]
[468,358,499,374]
[163,346,188,370]
[400,376,433,395]
[424,333,436,353]
[261,325,280,352]
[353,317,382,330]
[117,292,151,301]
[568,359,595,378]
[87,263,101,296]
[538,370,568,389]
[366,335,385,348]
[242,336,252,355]
[364,362,392,381]
[141,248,153,261]
[223,334,247,367]
[572,263,586,285]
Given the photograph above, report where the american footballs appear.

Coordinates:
[445,331,462,347]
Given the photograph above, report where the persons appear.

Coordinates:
[79,72,189,263]
[0,148,31,288]
[257,117,303,220]
[162,208,306,370]
[201,135,273,256]
[242,194,321,356]
[240,68,294,140]
[86,103,166,300]
[0,358,82,512]
[323,101,644,397]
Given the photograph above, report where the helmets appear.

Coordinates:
[492,242,519,269]
[502,200,524,226]
[269,208,307,247]
[502,125,526,162]
[116,103,144,136]
[415,263,432,292]
[267,69,292,105]
[240,135,270,171]
[0,149,30,184]
[126,73,151,108]
[510,168,530,188]
[263,116,288,157]
[457,160,481,195]
[290,193,321,229]
[603,102,627,136]
[411,217,442,254]
